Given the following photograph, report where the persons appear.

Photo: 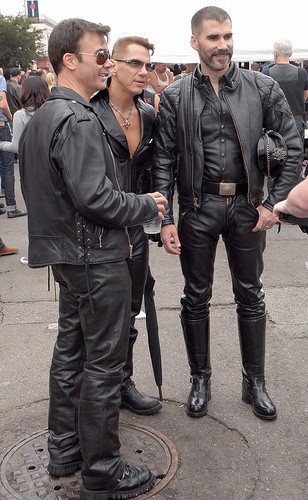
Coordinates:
[151,7,305,419]
[0,59,57,257]
[143,39,308,226]
[90,37,163,415]
[18,19,169,500]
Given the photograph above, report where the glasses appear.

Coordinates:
[114,57,157,74]
[71,49,110,66]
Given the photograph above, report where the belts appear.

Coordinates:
[203,181,248,195]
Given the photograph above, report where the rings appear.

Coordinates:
[265,225,270,229]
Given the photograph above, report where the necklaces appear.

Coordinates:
[109,101,133,130]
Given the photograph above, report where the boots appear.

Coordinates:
[178,311,212,419]
[237,313,277,420]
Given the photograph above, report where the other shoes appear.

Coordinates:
[120,379,162,416]
[0,209,6,216]
[80,465,156,500]
[0,244,18,256]
[46,458,83,476]
[7,209,28,218]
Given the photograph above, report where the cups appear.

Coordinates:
[143,219,162,234]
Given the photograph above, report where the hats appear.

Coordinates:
[257,128,287,180]
